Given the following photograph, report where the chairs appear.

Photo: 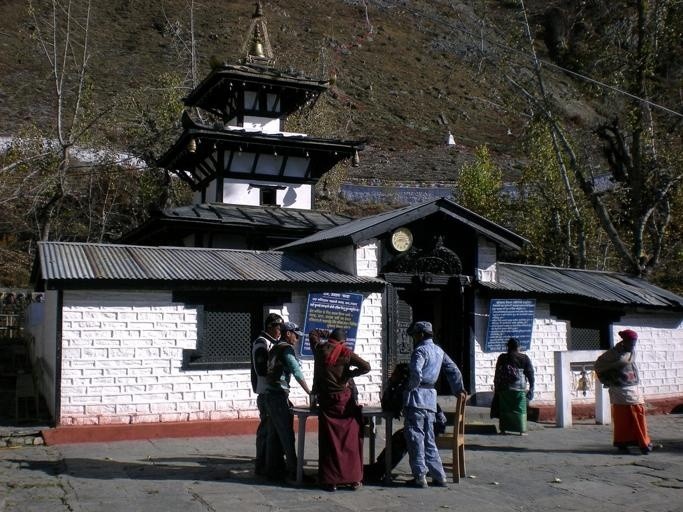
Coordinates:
[434,391,468,483]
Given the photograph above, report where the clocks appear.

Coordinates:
[390,226,414,253]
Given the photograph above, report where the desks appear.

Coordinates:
[287,405,394,482]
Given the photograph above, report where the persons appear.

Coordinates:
[261,322,312,483]
[251,314,294,479]
[403,321,468,489]
[309,327,371,491]
[363,363,448,480]
[493,337,534,437]
[595,330,663,456]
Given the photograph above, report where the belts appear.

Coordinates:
[416,382,436,389]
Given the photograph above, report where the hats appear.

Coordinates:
[281,322,305,337]
[618,330,637,339]
[406,321,432,333]
[265,313,283,327]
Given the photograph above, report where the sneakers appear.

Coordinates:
[344,483,362,490]
[322,484,336,492]
[290,470,316,483]
[405,475,428,489]
[616,447,629,453]
[500,431,507,434]
[520,432,527,435]
[426,476,448,487]
[643,443,653,454]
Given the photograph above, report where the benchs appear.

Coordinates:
[15,355,44,424]
[13,332,35,369]
